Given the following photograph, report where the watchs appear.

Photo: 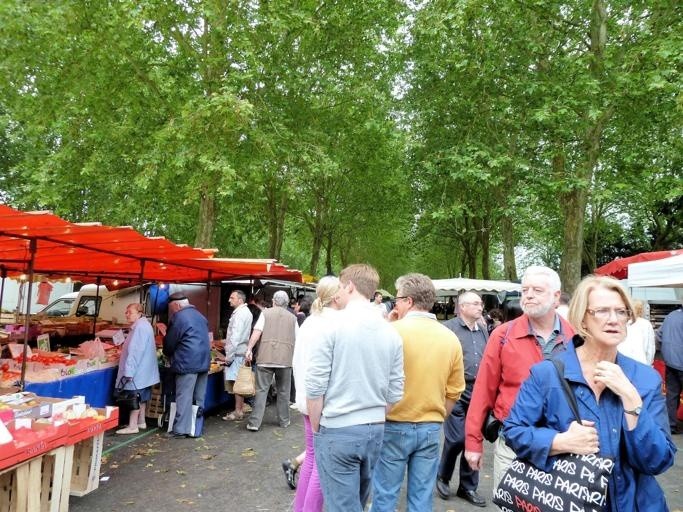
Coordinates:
[622,402,644,416]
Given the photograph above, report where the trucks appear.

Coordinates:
[70,282,321,330]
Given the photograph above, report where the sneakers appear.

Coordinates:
[134,422,147,429]
[281,458,297,490]
[245,422,261,433]
[222,413,244,420]
[116,426,139,436]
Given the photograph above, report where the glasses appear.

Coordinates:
[461,302,485,309]
[585,307,633,319]
[390,295,407,302]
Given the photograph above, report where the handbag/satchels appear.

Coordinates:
[116,376,141,410]
[232,358,255,397]
[492,359,616,512]
[165,402,199,436]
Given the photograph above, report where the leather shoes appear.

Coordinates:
[456,485,487,506]
[436,474,451,498]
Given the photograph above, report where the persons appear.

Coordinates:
[157,291,210,438]
[281,449,309,489]
[619,297,656,367]
[503,276,678,511]
[655,303,683,435]
[223,290,252,422]
[114,304,159,434]
[372,272,466,511]
[292,276,342,512]
[304,264,404,512]
[464,266,574,499]
[370,291,388,320]
[436,291,490,507]
[245,291,300,431]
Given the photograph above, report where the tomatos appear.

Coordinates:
[12,350,78,369]
[0,362,10,373]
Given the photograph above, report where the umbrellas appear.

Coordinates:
[418,277,523,291]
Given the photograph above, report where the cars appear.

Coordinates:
[33,294,78,319]
